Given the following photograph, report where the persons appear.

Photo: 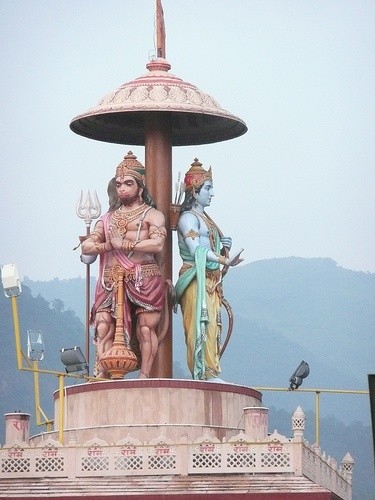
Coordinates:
[79,152,174,379]
[170,159,244,384]
